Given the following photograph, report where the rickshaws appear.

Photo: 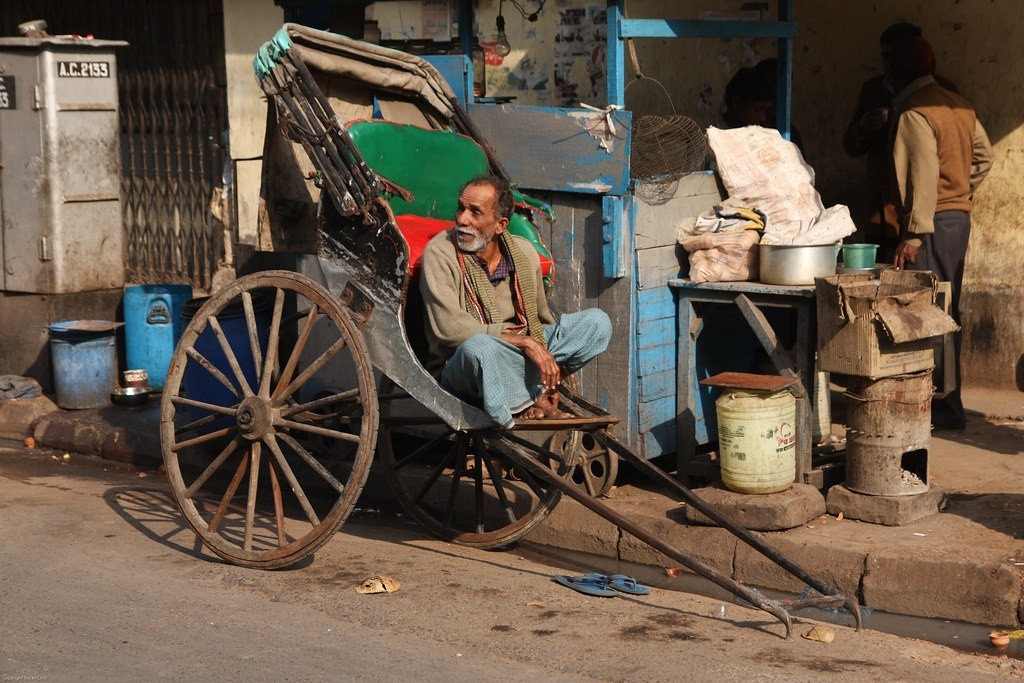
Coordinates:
[160,22,863,641]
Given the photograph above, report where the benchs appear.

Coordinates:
[318,118,552,282]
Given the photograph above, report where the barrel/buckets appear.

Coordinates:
[48,320,115,409]
[236,251,301,401]
[179,293,275,440]
[124,284,193,393]
[716,388,796,494]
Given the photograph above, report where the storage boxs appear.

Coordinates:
[812,268,960,382]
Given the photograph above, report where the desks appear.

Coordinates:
[668,278,954,443]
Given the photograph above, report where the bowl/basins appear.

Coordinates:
[754,242,838,285]
[112,387,154,406]
[840,244,880,268]
[123,369,148,387]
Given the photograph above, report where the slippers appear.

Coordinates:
[555,575,618,597]
[588,574,650,594]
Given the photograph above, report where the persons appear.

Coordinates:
[845,24,995,434]
[419,175,614,430]
[715,58,806,159]
